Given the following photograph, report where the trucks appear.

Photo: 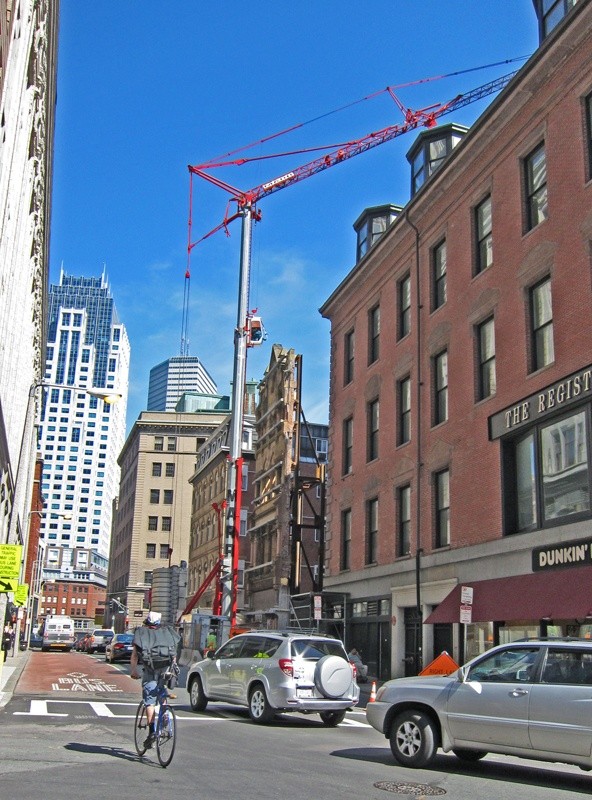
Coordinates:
[42,615,76,653]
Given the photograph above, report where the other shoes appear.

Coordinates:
[161,713,170,730]
[144,732,159,748]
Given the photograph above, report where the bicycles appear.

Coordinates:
[130,673,179,768]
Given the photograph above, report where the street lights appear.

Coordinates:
[12,510,74,658]
[23,557,58,643]
[0,382,122,654]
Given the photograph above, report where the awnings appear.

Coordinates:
[424,566,592,624]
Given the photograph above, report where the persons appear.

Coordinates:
[348,649,362,676]
[131,612,177,748]
[4,622,16,647]
[206,628,217,650]
[177,628,184,667]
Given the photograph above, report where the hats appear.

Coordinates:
[146,611,162,626]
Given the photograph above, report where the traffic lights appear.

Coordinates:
[109,601,113,609]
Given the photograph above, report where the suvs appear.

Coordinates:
[184,628,361,726]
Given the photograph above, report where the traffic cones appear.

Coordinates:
[368,681,377,703]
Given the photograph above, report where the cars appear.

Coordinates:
[364,634,592,770]
[105,634,134,665]
[74,629,117,654]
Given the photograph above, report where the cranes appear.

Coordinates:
[185,49,540,662]
